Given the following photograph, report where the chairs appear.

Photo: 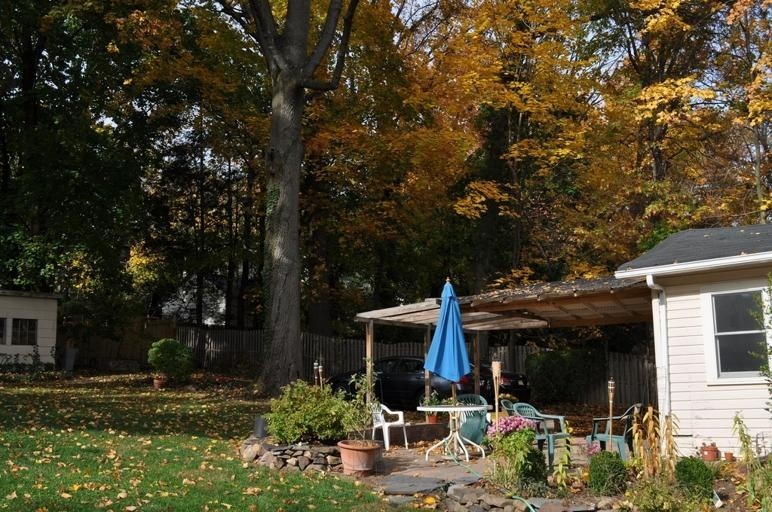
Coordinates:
[456,394,488,445]
[369,402,408,451]
[586,404,644,460]
[514,402,571,470]
[501,399,514,417]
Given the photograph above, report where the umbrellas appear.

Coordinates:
[422,276,473,455]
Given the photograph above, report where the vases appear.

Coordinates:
[724,451,734,462]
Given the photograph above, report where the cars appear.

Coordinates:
[325,356,533,411]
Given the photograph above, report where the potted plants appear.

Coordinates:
[147,338,195,389]
[330,358,380,476]
[700,442,719,462]
